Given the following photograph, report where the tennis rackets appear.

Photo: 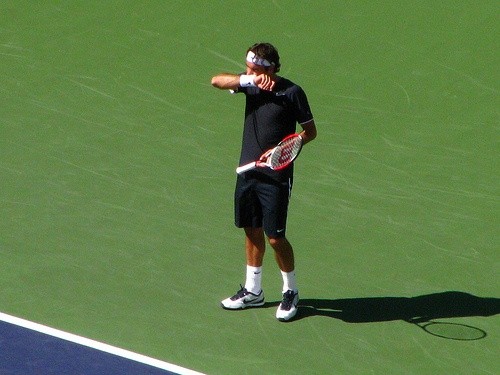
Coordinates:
[236,134,304,175]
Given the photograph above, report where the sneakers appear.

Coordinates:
[276,288,300,321]
[221,284,265,310]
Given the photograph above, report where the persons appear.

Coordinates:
[211,42,317,321]
